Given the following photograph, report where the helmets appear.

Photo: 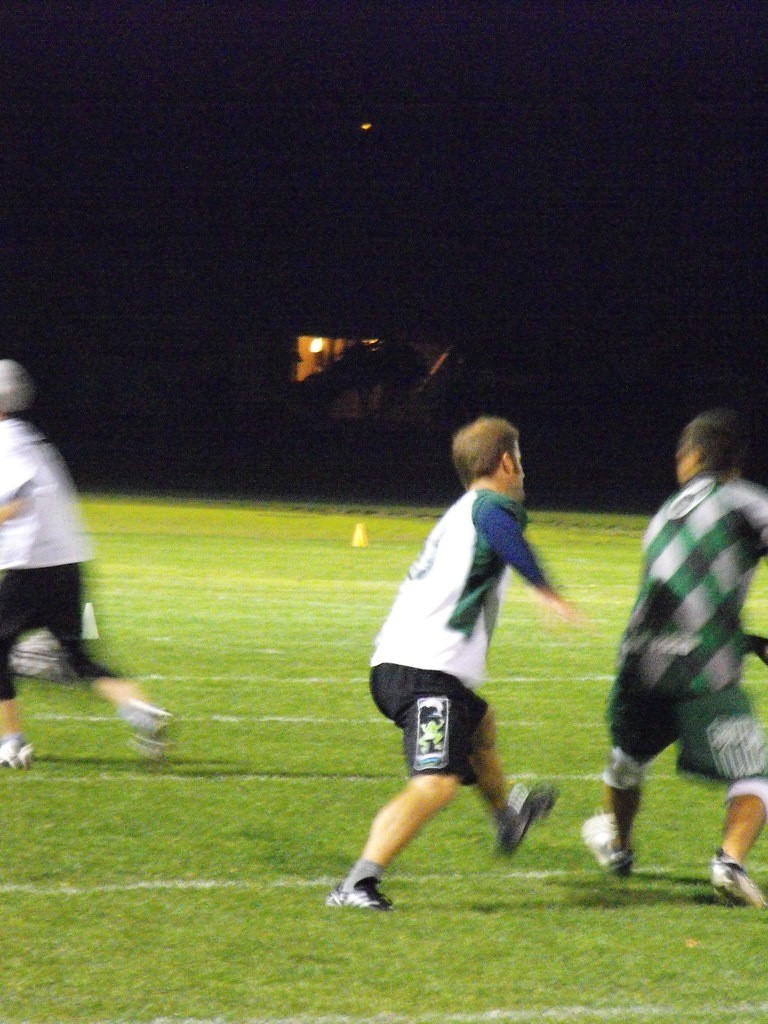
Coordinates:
[0,359,36,411]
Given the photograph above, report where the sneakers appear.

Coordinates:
[326,876,392,912]
[496,782,560,854]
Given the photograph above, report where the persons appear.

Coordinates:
[577,405,768,909]
[327,415,582,912]
[0,360,174,769]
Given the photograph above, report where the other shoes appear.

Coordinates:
[116,701,172,761]
[0,739,35,769]
[710,848,764,907]
[581,815,634,878]
